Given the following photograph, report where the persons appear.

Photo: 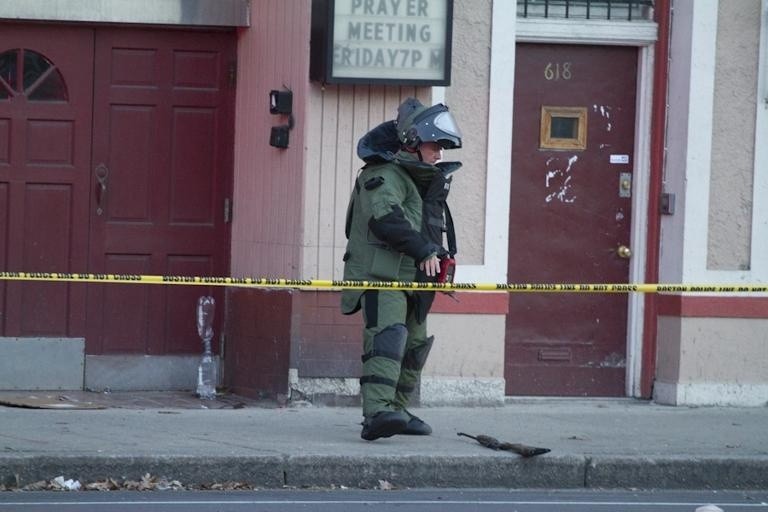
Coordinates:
[341,97,462,440]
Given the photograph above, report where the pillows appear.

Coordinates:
[393,99,463,151]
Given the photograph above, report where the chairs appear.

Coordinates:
[360,411,432,441]
